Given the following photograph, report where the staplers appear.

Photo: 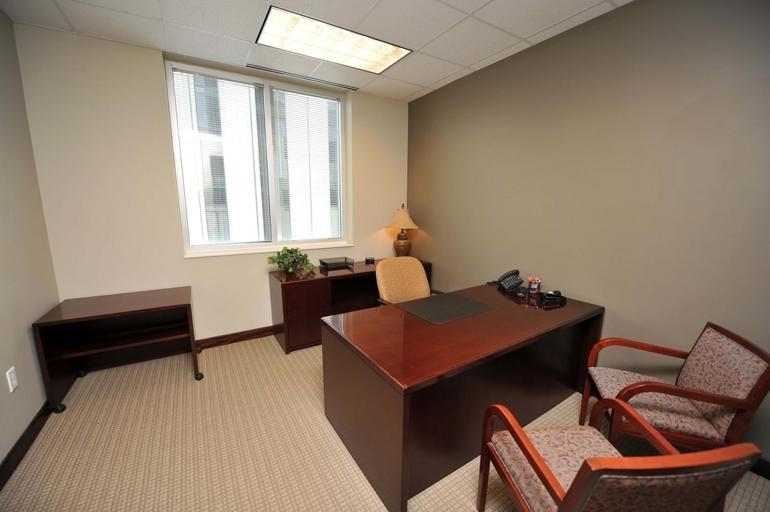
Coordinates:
[542,296,566,305]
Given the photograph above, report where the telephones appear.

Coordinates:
[497,270,523,291]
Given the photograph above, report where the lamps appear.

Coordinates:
[390,205,418,255]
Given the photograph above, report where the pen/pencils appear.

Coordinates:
[528,273,541,295]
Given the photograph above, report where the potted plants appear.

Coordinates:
[269,248,314,277]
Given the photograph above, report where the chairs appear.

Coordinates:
[476,399,762,512]
[579,324,769,446]
[376,258,445,304]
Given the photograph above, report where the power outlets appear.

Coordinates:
[6,366,18,393]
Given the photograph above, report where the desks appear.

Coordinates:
[34,285,204,412]
[269,255,430,353]
[321,282,605,512]
